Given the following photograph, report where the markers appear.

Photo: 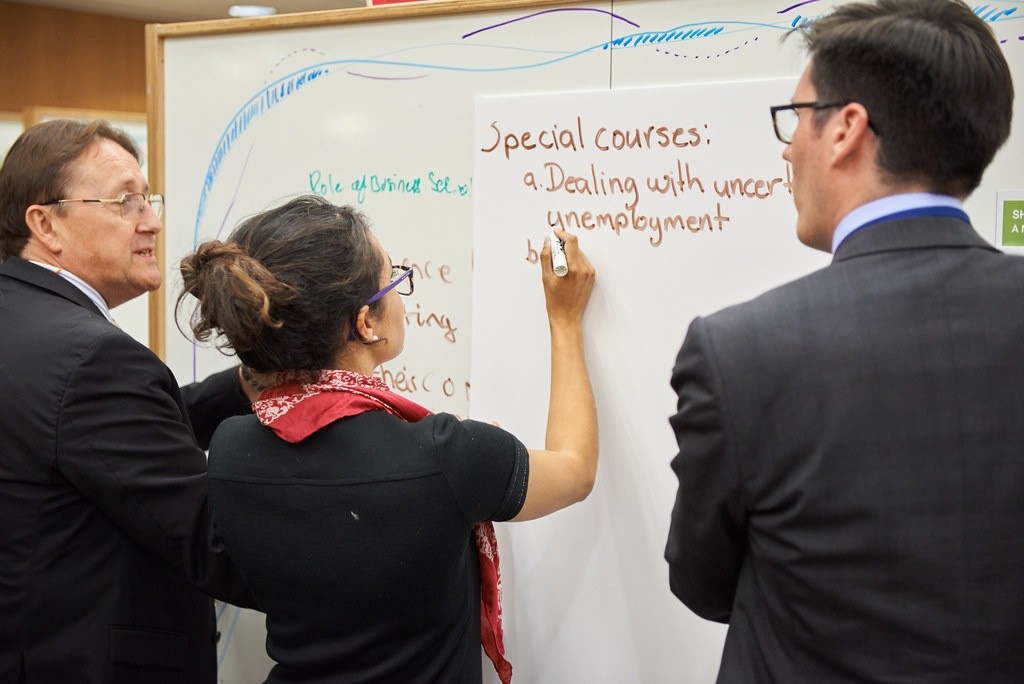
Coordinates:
[548,224,570,278]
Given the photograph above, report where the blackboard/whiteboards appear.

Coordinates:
[146,0,1024,684]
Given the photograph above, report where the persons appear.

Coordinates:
[664,0,1024,684]
[0,119,257,684]
[174,196,599,684]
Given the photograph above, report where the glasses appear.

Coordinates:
[348,265,414,341]
[770,102,880,145]
[41,192,164,220]
